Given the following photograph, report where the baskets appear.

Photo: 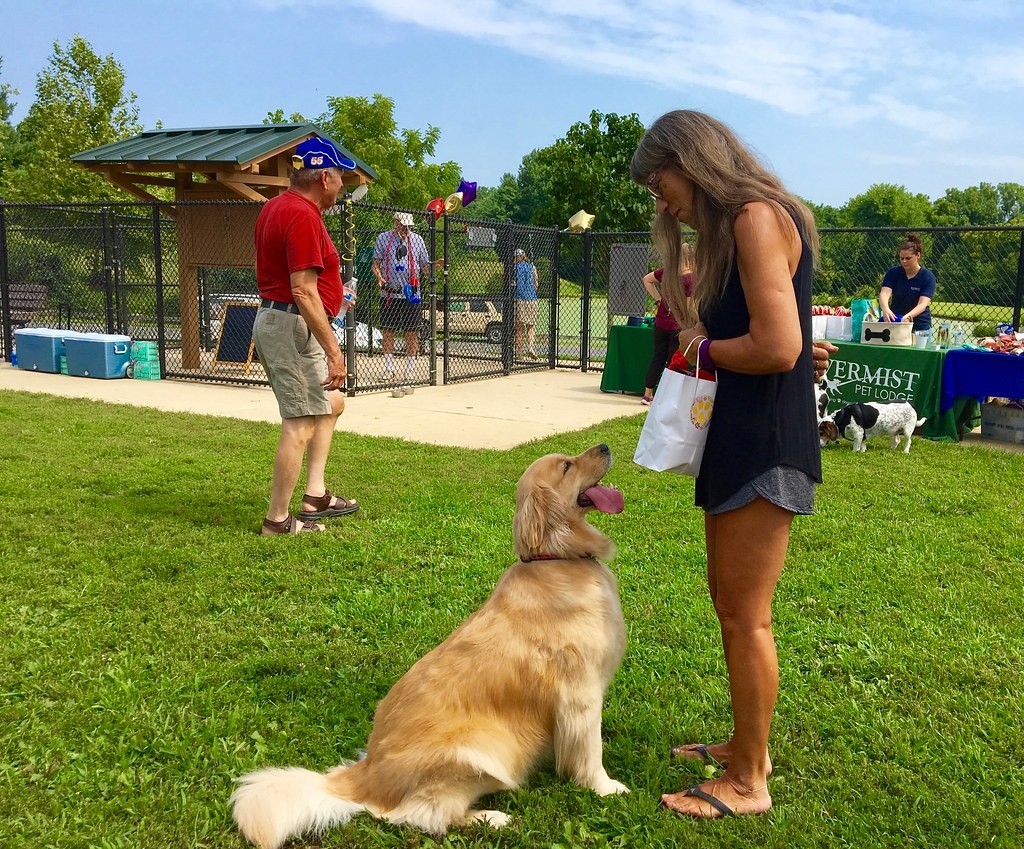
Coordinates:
[860,312,914,346]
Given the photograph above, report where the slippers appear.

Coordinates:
[657,785,764,818]
[668,745,731,772]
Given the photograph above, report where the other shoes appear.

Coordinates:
[512,355,524,363]
[403,370,414,382]
[377,370,394,383]
[527,348,539,361]
[640,395,654,405]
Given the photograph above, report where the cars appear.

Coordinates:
[196,293,383,354]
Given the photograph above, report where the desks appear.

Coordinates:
[598,324,679,397]
[813,337,981,442]
[940,349,1024,442]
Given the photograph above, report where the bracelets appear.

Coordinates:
[698,340,715,370]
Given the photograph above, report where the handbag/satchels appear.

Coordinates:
[632,336,719,478]
[812,305,854,341]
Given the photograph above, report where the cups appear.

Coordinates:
[916,337,927,348]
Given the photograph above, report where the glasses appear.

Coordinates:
[647,156,670,202]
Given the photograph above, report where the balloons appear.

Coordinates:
[568,209,595,234]
[444,192,463,217]
[456,180,477,207]
[425,198,445,223]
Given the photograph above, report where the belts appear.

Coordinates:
[260,298,334,325]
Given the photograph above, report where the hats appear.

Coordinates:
[513,249,523,256]
[393,211,415,226]
[291,137,357,171]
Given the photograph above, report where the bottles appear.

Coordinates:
[334,277,357,320]
[954,323,962,347]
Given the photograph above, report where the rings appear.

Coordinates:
[815,360,819,370]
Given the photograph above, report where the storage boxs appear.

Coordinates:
[63,333,134,379]
[132,360,161,380]
[851,298,881,342]
[130,341,158,361]
[59,356,69,375]
[812,315,827,339]
[16,327,79,374]
[826,315,852,340]
[980,403,1024,445]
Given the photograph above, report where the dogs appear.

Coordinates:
[813,380,928,453]
[227,444,627,849]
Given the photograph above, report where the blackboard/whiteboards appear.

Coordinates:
[213,302,262,366]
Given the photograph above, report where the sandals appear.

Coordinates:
[260,515,324,535]
[298,489,360,521]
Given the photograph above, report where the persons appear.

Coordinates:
[643,242,697,405]
[372,211,445,381]
[253,136,359,536]
[513,249,538,360]
[880,234,935,343]
[631,110,838,819]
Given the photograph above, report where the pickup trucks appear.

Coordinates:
[421,296,502,344]
